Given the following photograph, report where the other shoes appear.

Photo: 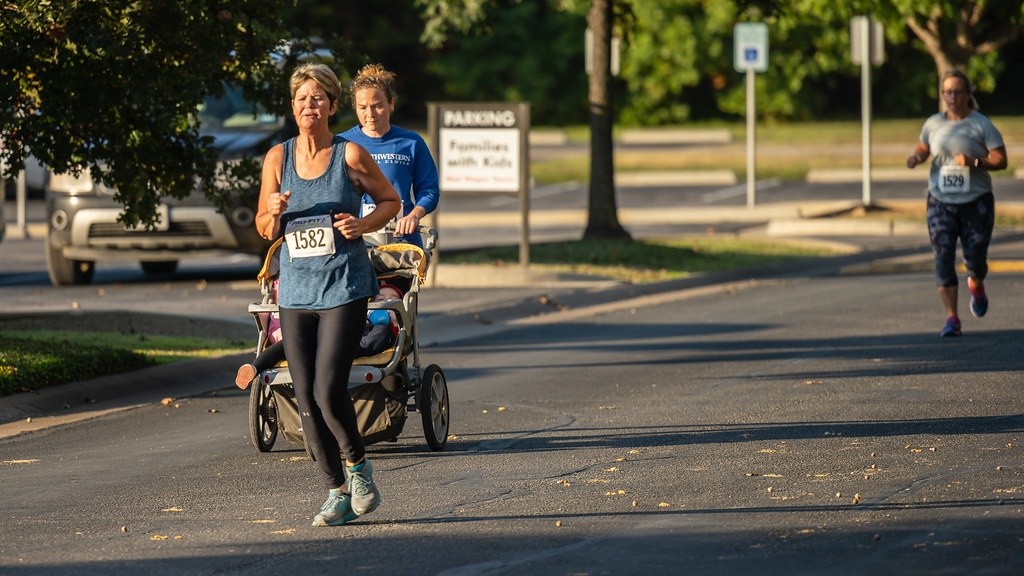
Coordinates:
[236,364,257,389]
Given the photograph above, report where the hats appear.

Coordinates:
[379,283,405,297]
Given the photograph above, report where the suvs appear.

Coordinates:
[0,38,354,285]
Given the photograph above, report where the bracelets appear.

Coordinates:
[917,154,922,164]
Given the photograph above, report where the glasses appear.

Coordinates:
[944,90,967,97]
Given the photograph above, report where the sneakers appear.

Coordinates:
[941,314,963,338]
[345,458,380,515]
[311,489,358,527]
[967,276,990,319]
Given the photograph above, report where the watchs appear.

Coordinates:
[973,158,982,168]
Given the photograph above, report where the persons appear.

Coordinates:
[907,68,1008,337]
[254,63,401,527]
[236,66,429,440]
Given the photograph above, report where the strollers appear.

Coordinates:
[248,221,450,452]
